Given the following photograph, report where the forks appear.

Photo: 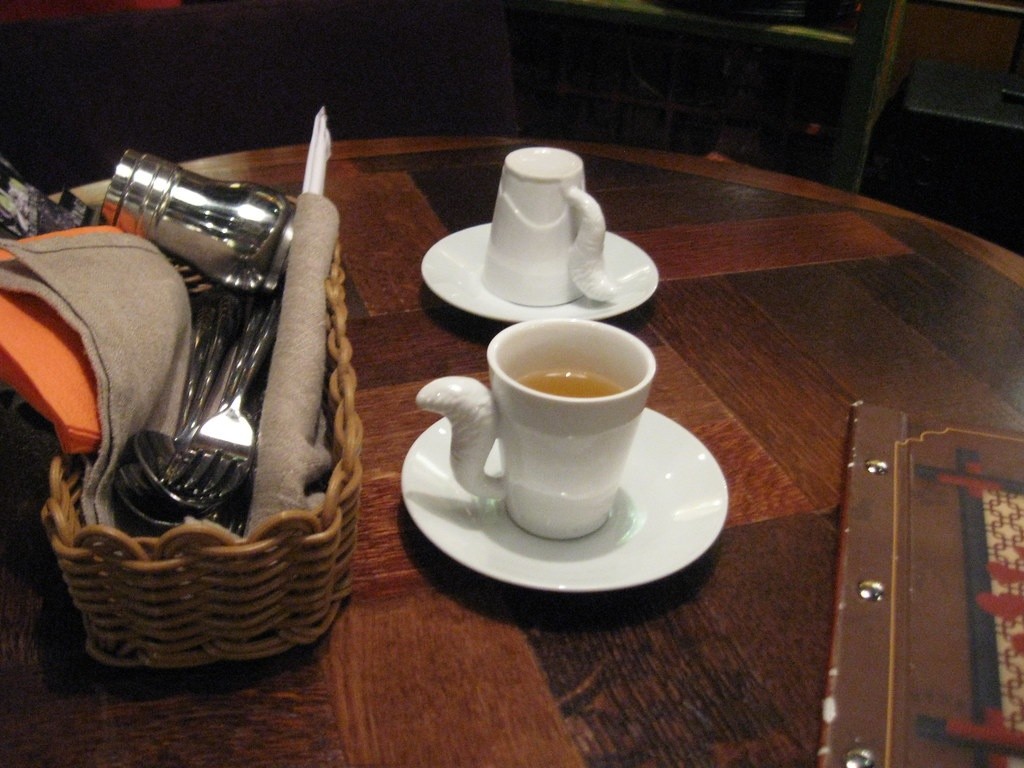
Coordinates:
[160,297,280,498]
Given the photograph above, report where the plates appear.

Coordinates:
[401,406,729,592]
[421,222,660,322]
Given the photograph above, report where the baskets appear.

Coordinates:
[42,196,362,668]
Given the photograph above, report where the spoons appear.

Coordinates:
[114,286,281,535]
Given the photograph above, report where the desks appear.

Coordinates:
[0,134,1024,768]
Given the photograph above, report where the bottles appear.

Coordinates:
[99,148,301,291]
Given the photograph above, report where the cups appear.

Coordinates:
[415,319,657,539]
[483,147,616,307]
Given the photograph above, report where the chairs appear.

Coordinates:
[0,0,517,194]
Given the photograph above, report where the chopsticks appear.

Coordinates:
[304,113,327,194]
